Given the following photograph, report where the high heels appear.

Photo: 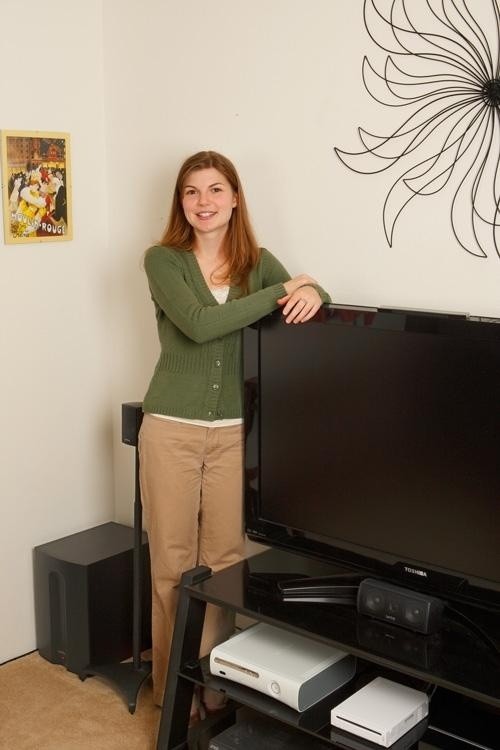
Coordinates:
[189,685,227,722]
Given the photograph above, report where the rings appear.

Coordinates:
[300,298,307,305]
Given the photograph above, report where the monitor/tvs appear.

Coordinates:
[244,301,500,653]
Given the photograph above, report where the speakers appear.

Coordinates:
[33,521,152,676]
[122,402,144,446]
[356,577,443,635]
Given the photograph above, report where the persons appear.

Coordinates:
[136,149,334,729]
[8,161,67,237]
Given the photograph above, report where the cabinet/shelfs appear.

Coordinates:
[152,545,500,750]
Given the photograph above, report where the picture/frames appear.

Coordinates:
[1,129,74,247]
